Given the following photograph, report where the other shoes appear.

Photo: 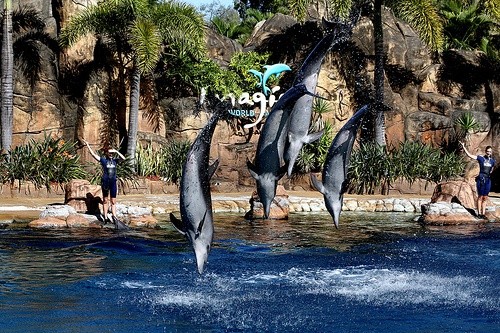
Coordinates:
[482,214,488,219]
[477,214,482,218]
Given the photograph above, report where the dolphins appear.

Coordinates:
[245,30,368,231]
[168,105,223,275]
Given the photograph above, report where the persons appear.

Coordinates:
[81,139,126,223]
[459,141,495,219]
